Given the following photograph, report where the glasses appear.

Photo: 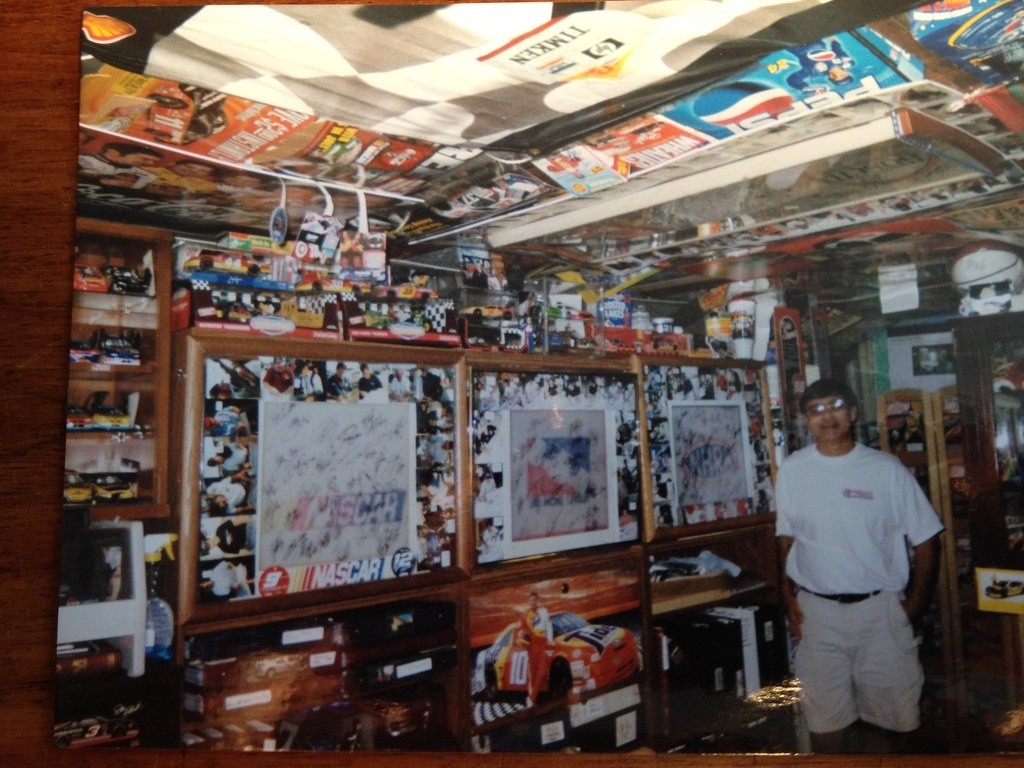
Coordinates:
[807,397,849,417]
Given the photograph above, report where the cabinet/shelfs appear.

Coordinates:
[70,216,172,524]
[151,326,799,752]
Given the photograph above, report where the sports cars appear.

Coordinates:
[476,609,643,704]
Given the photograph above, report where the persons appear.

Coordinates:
[502,373,637,410]
[203,405,247,436]
[296,361,457,404]
[419,405,454,571]
[264,359,294,395]
[77,132,391,237]
[518,593,554,708]
[210,368,261,398]
[615,411,637,526]
[546,151,586,181]
[202,472,255,516]
[340,231,365,269]
[642,365,775,528]
[472,369,504,563]
[786,36,855,94]
[204,517,247,554]
[207,439,251,472]
[199,560,241,598]
[472,263,501,290]
[774,379,947,754]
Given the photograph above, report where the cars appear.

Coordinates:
[73,261,151,295]
[184,250,272,279]
[62,468,136,506]
[67,402,134,432]
[71,332,143,369]
[985,580,1024,599]
[459,304,516,321]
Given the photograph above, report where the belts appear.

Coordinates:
[796,586,881,604]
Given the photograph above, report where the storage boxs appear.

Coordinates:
[175,231,694,356]
